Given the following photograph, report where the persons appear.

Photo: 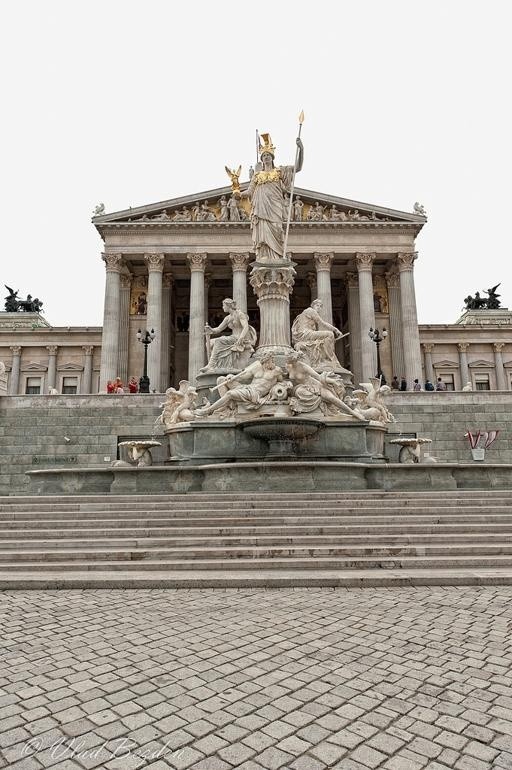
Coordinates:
[8,291,18,301]
[138,295,147,314]
[487,288,499,299]
[248,165,254,181]
[376,370,386,386]
[114,377,124,393]
[400,376,407,390]
[129,376,139,393]
[234,137,304,271]
[107,381,114,394]
[158,297,396,424]
[128,445,154,466]
[128,191,382,223]
[413,379,421,391]
[435,378,446,391]
[391,376,399,389]
[399,444,421,464]
[424,380,434,391]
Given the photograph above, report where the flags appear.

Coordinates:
[257,135,262,154]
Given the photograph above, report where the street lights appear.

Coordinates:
[136,327,155,393]
[368,326,388,386]
